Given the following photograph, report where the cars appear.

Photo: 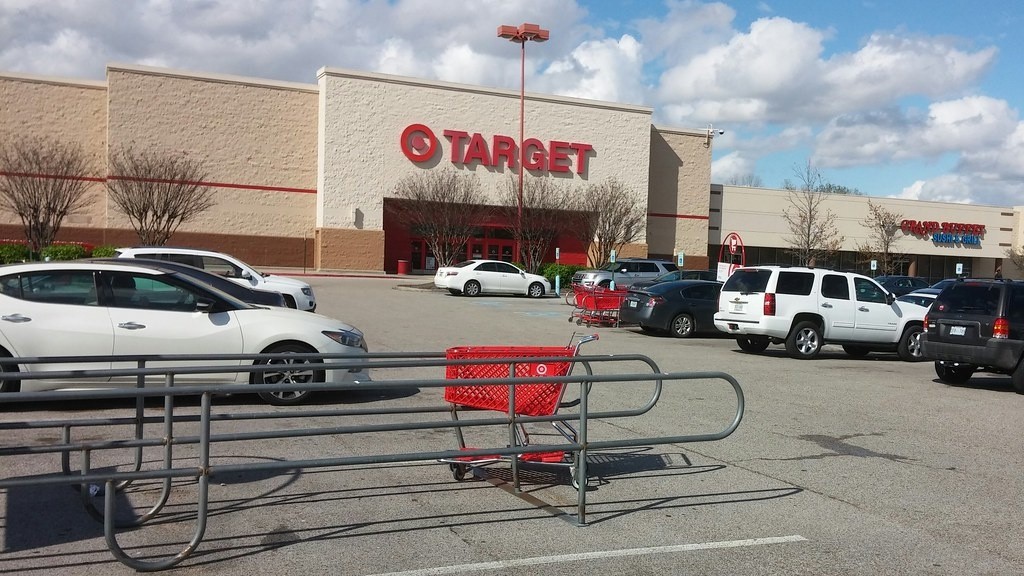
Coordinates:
[0,262,374,407]
[433,258,552,298]
[854,276,932,303]
[5,257,288,307]
[895,278,956,307]
[630,269,717,290]
[618,282,725,336]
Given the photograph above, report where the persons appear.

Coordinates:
[995,269,1002,278]
[112,275,138,302]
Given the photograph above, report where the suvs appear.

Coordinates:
[115,247,318,311]
[571,257,680,290]
[919,275,1024,395]
[714,264,931,362]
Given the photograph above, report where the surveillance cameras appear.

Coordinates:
[719,129,724,135]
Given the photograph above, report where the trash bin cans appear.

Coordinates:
[397,259,409,275]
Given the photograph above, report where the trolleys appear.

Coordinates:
[436,328,600,489]
[566,283,627,327]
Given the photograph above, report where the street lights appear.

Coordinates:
[498,23,549,260]
